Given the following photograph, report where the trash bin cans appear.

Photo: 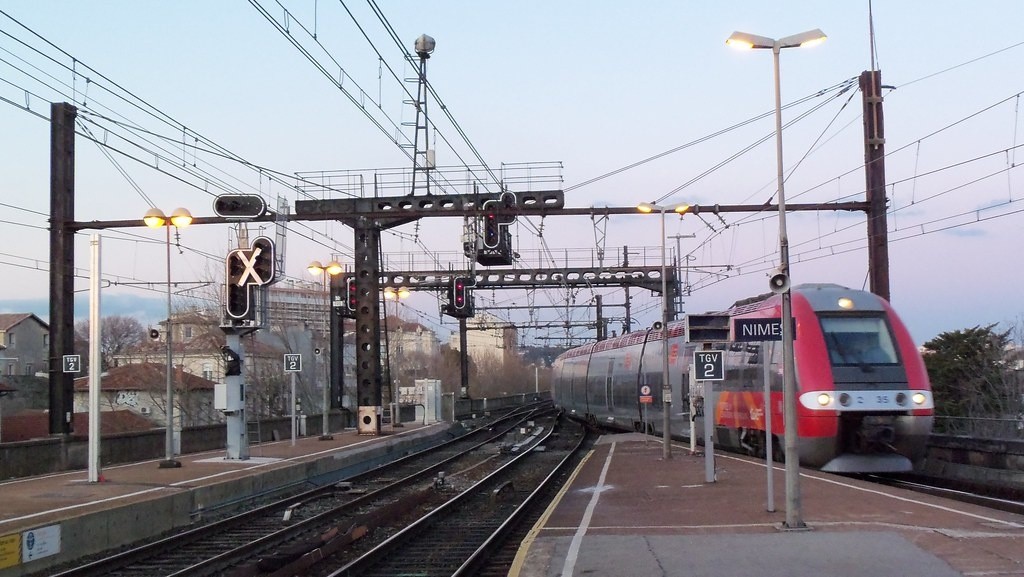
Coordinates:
[357,405,383,436]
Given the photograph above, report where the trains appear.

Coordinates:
[549,282,935,479]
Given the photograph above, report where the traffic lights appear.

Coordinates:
[347,278,356,312]
[482,191,518,250]
[454,275,477,310]
[213,192,268,219]
[223,236,276,322]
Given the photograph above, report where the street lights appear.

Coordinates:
[140,209,193,469]
[385,286,411,429]
[637,201,689,459]
[722,26,829,532]
[307,259,344,441]
